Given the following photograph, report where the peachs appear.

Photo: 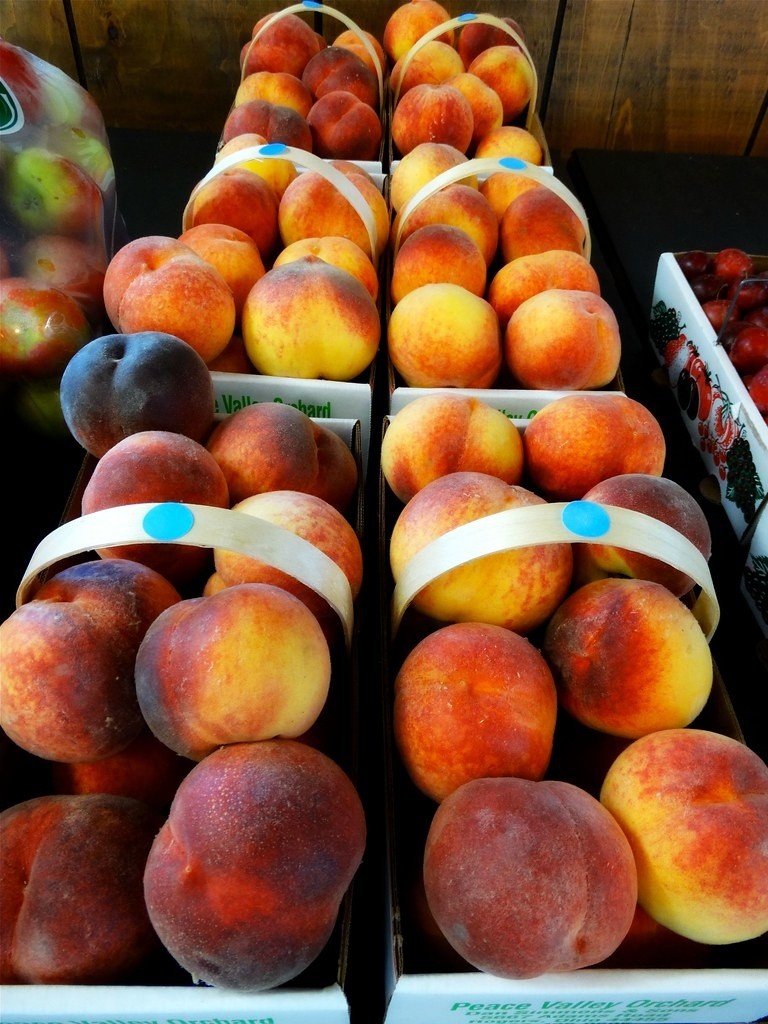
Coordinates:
[1,1,768,994]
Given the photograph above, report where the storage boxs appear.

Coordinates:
[648,249,768,641]
[386,65,554,177]
[0,415,363,1024]
[376,414,768,1024]
[209,168,389,490]
[215,46,387,173]
[382,174,628,419]
